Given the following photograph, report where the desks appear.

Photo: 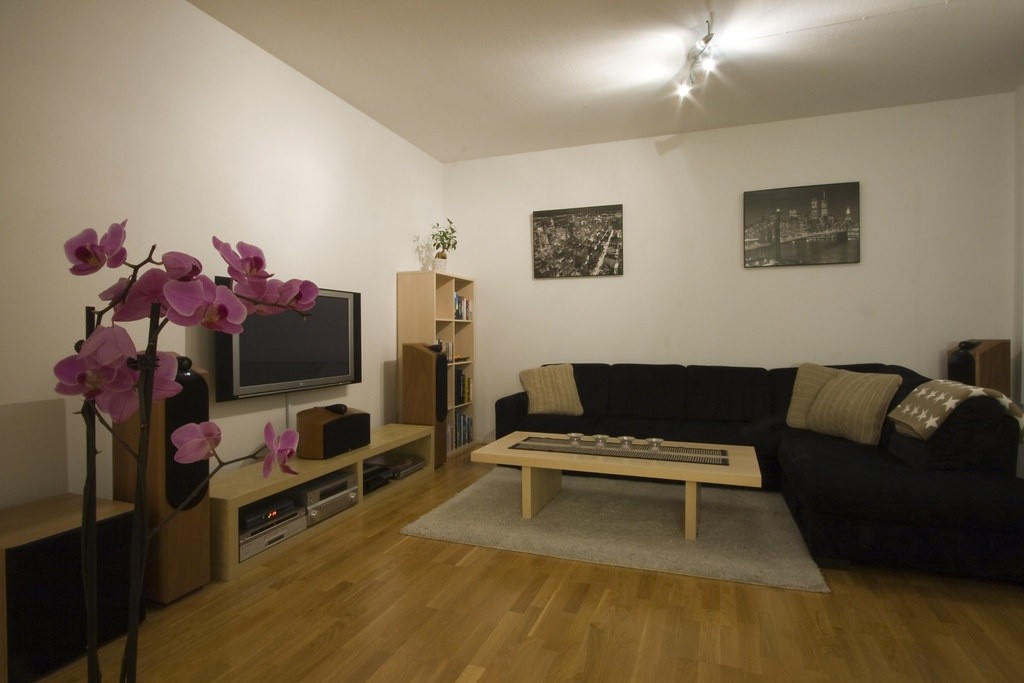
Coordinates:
[209,424,435,583]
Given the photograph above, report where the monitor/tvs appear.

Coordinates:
[213,276,362,402]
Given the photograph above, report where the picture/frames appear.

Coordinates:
[533,203,625,279]
[741,182,861,270]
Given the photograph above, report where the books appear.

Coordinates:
[437,339,453,363]
[455,410,473,450]
[455,369,471,405]
[455,295,472,320]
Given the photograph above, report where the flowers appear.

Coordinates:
[52,218,319,683]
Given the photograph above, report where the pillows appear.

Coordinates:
[787,362,839,430]
[807,371,902,445]
[520,364,584,416]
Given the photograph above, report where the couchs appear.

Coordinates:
[493,363,1024,586]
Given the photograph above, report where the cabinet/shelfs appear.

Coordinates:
[397,270,473,459]
[1,490,145,683]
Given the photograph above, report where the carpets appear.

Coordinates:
[401,468,830,595]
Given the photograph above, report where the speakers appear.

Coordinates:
[402,343,449,470]
[296,406,371,459]
[948,339,1011,399]
[112,353,210,604]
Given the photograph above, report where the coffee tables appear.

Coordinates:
[470,431,761,541]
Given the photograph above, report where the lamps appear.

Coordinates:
[676,19,715,102]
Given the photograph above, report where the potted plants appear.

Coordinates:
[431,219,457,271]
